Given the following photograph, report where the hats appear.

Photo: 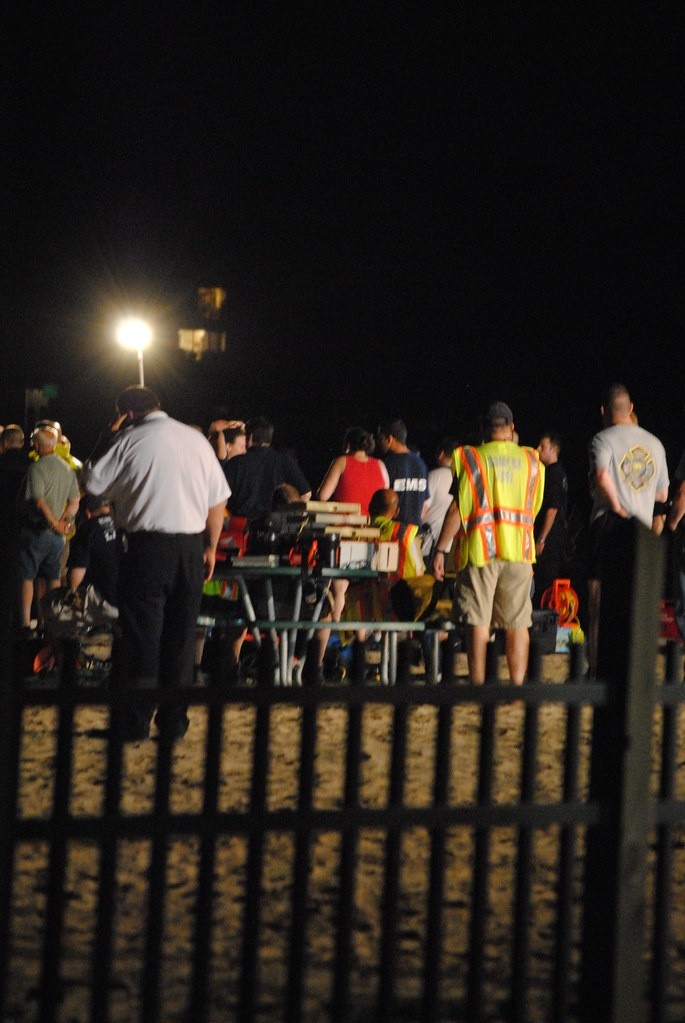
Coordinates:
[482,402,513,427]
[207,419,230,438]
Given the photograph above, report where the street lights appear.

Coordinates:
[113,316,154,388]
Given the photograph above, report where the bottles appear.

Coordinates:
[63,517,76,538]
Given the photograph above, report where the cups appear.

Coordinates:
[323,533,340,568]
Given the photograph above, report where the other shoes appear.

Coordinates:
[88,728,108,739]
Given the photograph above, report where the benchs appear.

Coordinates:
[223,621,425,685]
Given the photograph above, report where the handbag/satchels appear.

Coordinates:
[246,510,301,554]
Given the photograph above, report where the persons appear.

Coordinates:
[583,383,685,687]
[83,384,232,741]
[530,431,570,612]
[189,416,463,691]
[0,419,125,643]
[430,400,547,706]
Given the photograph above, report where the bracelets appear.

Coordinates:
[435,549,444,553]
[537,538,544,543]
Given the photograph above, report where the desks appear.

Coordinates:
[208,566,381,686]
